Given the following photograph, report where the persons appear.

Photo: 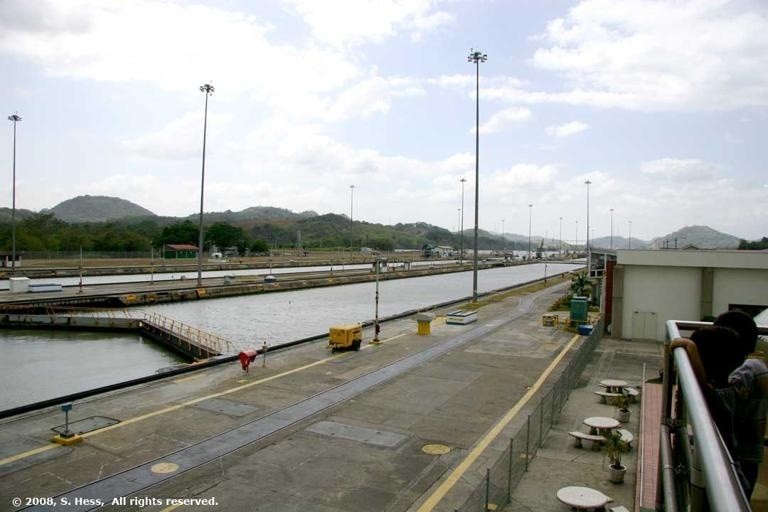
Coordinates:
[668,310,768,503]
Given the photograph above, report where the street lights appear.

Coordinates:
[347,179,634,269]
[196,80,215,287]
[6,111,24,273]
[463,49,492,304]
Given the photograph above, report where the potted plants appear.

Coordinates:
[604,433,633,484]
[570,270,593,304]
[613,394,632,421]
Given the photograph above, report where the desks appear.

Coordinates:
[554,487,607,511]
[600,379,628,392]
[583,418,621,434]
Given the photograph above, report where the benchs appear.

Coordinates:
[609,506,629,512]
[568,429,633,453]
[593,388,639,405]
